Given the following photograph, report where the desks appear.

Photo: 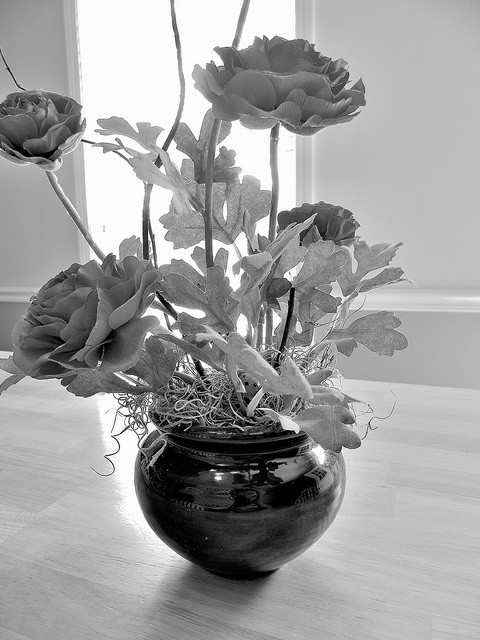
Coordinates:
[0,352,480,640]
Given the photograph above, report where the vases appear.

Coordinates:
[135,401,347,582]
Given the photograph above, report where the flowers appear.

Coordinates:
[0,1,414,475]
